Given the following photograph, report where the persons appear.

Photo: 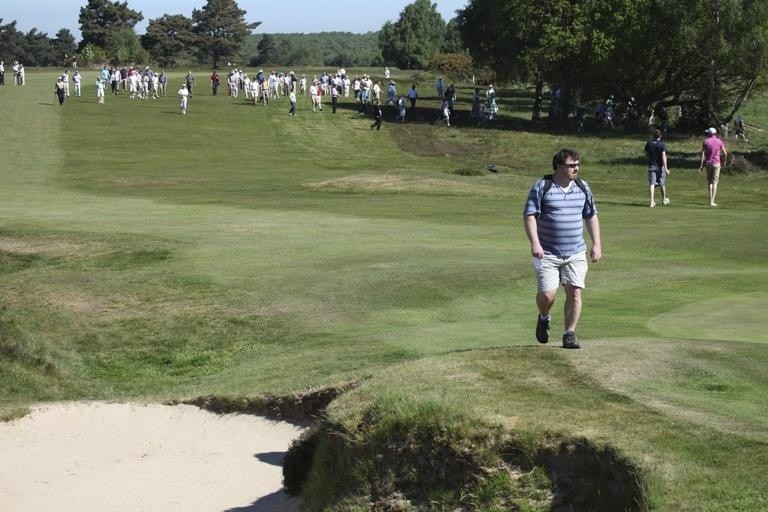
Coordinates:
[71,70,83,98]
[0,61,6,86]
[61,69,71,98]
[12,60,20,86]
[13,63,25,87]
[54,76,65,107]
[697,127,728,207]
[72,56,76,68]
[643,128,671,209]
[733,113,750,142]
[597,93,681,133]
[95,65,168,104]
[177,68,220,115]
[433,76,499,126]
[226,68,308,117]
[309,66,418,132]
[524,148,603,349]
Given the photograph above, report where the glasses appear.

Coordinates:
[560,164,579,168]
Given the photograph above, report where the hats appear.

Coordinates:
[706,128,717,134]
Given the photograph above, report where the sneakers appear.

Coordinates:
[536,313,551,343]
[563,334,580,348]
[650,202,656,207]
[662,197,669,204]
